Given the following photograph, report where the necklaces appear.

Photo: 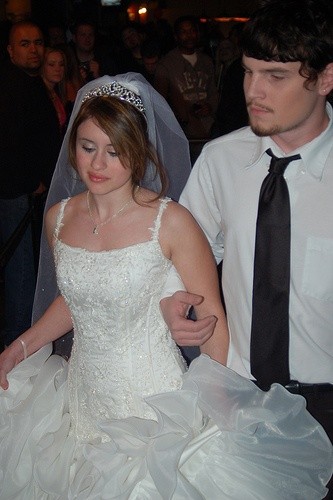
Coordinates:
[86,186,140,234]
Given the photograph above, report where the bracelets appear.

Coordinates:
[17,337,27,361]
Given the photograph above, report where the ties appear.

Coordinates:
[249,149,303,392]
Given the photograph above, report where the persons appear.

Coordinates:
[68,19,107,84]
[0,0,32,58]
[153,14,220,139]
[118,26,145,73]
[39,47,77,143]
[0,73,231,500]
[42,25,67,49]
[0,17,58,354]
[139,41,162,86]
[207,21,231,64]
[161,0,333,500]
[214,40,247,137]
